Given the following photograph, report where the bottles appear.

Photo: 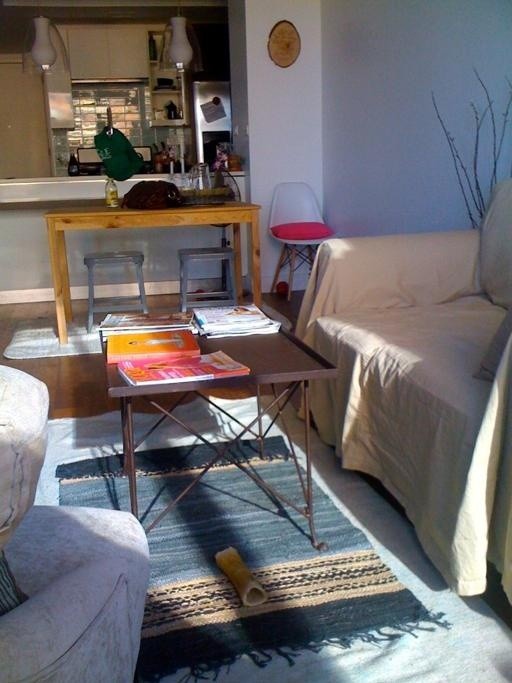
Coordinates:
[67,149,80,177]
[106,177,118,209]
[170,163,212,205]
[148,32,158,61]
[166,101,185,117]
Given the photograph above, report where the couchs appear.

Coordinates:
[292,178,511,632]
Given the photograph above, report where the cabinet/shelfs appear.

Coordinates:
[40,23,75,130]
[147,23,192,127]
[67,23,149,84]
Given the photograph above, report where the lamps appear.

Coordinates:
[21,0,69,74]
[158,1,202,72]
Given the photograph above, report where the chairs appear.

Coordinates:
[267,182,335,302]
[0,364,149,683]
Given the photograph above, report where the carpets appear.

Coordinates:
[54,434,452,683]
[33,395,512,683]
[3,297,291,360]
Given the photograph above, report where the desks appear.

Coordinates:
[45,202,262,345]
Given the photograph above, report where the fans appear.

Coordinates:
[206,168,248,299]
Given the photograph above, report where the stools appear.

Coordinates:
[83,251,150,334]
[179,246,238,312]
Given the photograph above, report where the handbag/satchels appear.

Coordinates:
[121,180,184,210]
[94,126,147,181]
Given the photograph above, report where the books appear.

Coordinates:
[117,349,251,390]
[94,303,283,340]
[106,329,201,365]
